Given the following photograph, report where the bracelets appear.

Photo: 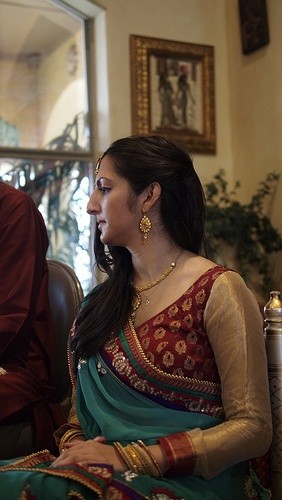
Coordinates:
[113,439,156,481]
[59,424,86,456]
[137,438,165,480]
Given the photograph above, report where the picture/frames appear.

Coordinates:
[129,34,217,156]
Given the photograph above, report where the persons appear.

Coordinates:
[1,133,281,500]
[0,179,71,462]
[156,70,197,129]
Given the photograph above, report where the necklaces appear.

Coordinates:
[128,248,184,321]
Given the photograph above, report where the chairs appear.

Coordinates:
[0,258,84,453]
